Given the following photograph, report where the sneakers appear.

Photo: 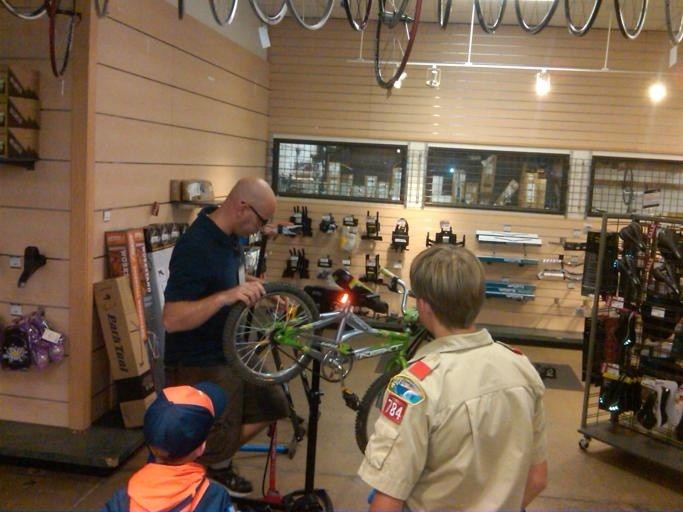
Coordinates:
[204,460,253,494]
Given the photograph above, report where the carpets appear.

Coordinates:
[374,346,584,392]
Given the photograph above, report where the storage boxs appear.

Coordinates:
[92,221,193,430]
[325,153,547,213]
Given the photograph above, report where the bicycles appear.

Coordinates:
[224,267,435,455]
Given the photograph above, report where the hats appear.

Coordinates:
[142,381,227,458]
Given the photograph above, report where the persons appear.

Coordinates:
[356,242,552,512]
[160,174,292,496]
[98,379,241,512]
